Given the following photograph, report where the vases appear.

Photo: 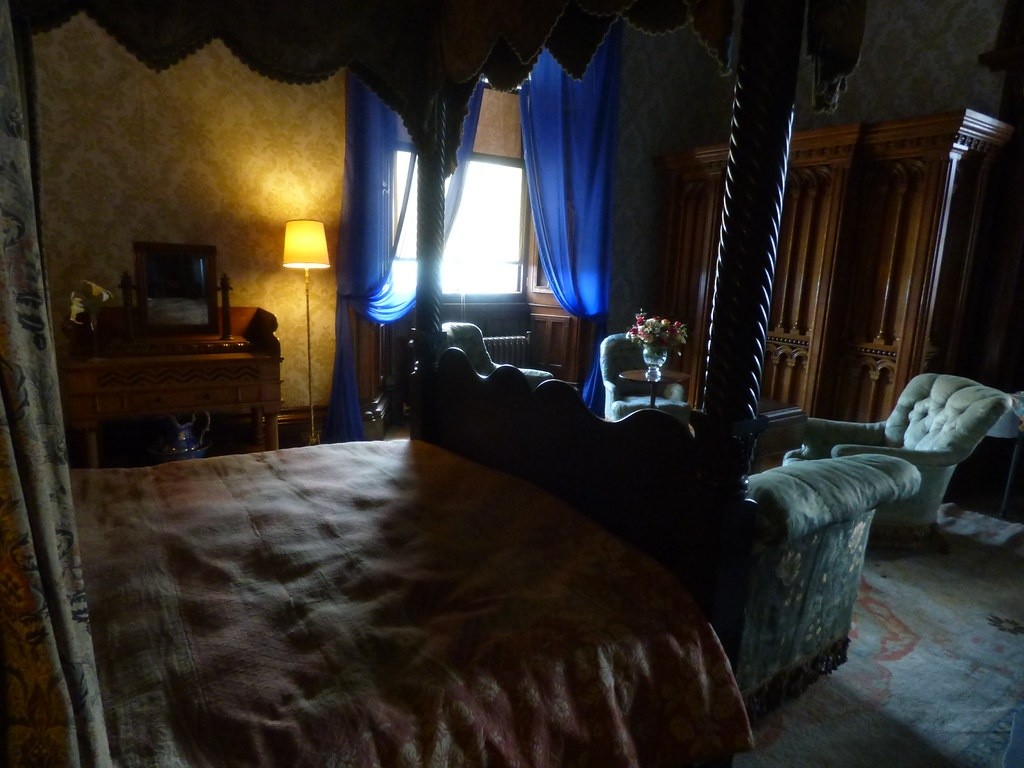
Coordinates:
[642,346,668,378]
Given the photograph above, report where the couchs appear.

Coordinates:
[438,322,921,719]
[600,334,691,430]
[780,372,1012,547]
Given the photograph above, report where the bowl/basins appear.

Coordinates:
[146,439,213,460]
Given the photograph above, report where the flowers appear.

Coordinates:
[626,307,687,350]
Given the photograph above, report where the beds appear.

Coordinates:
[70,439,754,768]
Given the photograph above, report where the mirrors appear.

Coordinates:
[132,240,219,337]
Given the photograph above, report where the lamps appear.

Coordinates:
[283,219,330,445]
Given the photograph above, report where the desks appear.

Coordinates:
[51,307,283,468]
